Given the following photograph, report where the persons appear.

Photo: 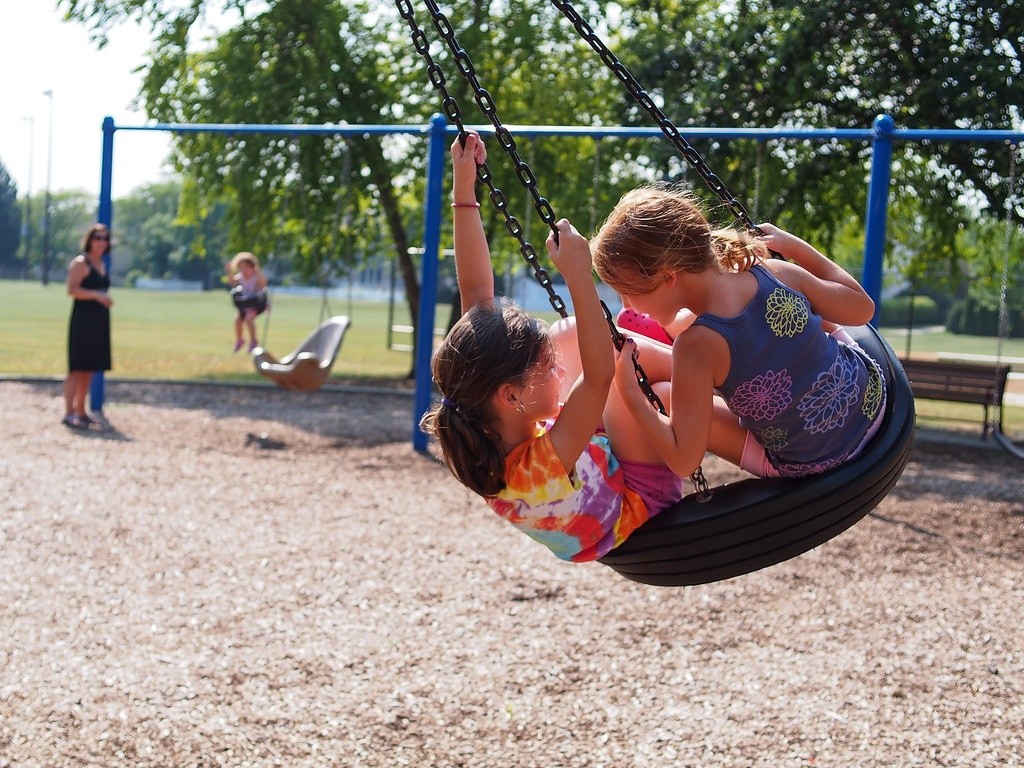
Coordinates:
[418,130,673,563]
[587,182,887,479]
[225,252,269,353]
[61,224,113,429]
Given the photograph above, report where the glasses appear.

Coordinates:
[92,234,108,241]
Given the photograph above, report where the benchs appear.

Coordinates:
[900,357,1011,446]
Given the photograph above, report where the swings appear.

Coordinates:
[395,0,915,589]
[250,132,352,392]
[990,136,1024,462]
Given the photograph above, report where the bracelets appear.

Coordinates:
[450,202,480,207]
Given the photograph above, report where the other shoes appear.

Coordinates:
[64,415,101,431]
[248,342,258,352]
[235,340,245,351]
